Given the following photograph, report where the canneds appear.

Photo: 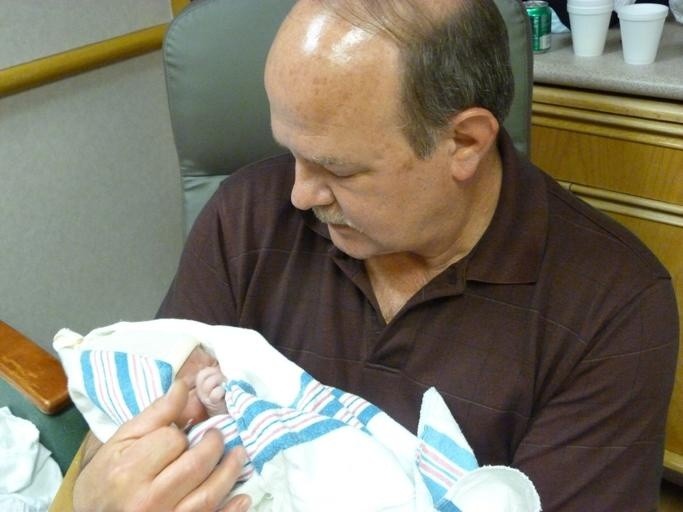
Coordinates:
[524,0,552,55]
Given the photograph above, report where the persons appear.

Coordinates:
[98,319,543,512]
[51,0,680,512]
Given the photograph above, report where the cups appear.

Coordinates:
[616,4,669,65]
[566,1,614,57]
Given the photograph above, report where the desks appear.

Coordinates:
[533,24,683,485]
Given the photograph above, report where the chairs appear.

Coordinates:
[161,4,530,263]
[1,317,91,476]
